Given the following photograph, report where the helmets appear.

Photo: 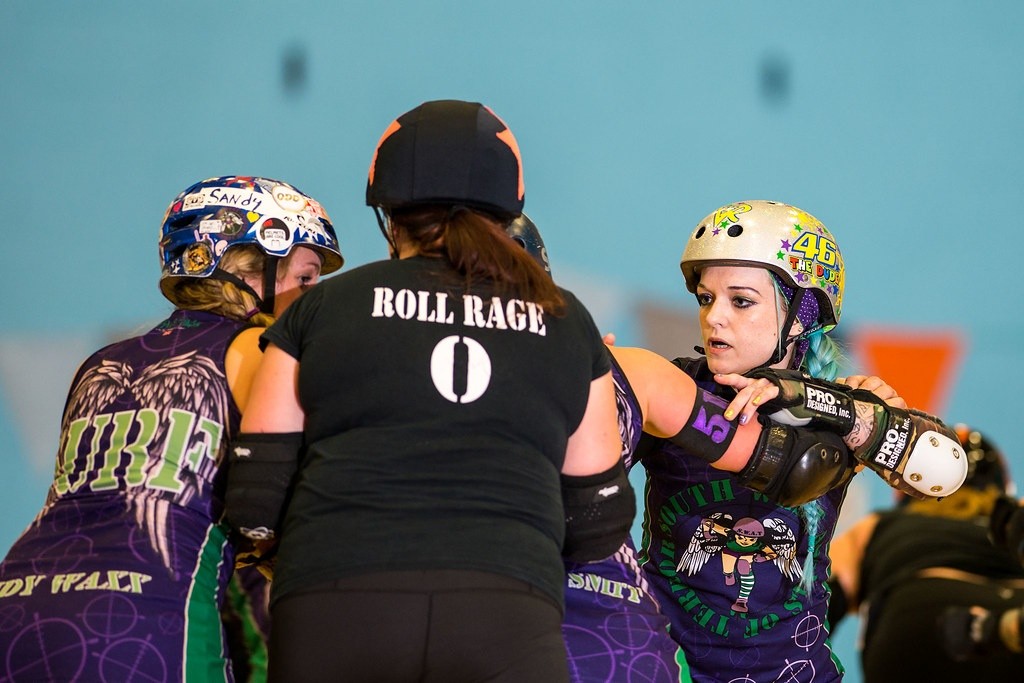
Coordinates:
[156,175,346,323]
[680,199,844,372]
[506,214,551,287]
[366,98,524,257]
[895,423,1011,517]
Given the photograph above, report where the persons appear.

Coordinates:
[226,98,637,683]
[504,215,910,682]
[0,175,345,683]
[600,199,967,682]
[830,421,1024,682]
